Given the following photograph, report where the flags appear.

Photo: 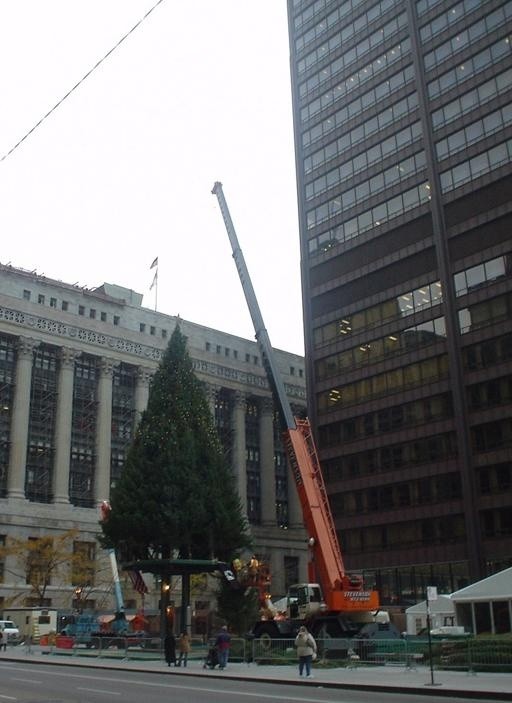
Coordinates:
[149,274,158,290]
[149,258,158,270]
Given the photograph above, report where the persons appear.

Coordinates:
[0,627,8,652]
[232,555,242,576]
[164,631,177,666]
[214,625,232,670]
[249,556,259,579]
[294,624,318,677]
[176,629,191,668]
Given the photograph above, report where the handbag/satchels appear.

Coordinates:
[307,639,314,648]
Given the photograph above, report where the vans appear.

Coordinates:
[0,620,20,646]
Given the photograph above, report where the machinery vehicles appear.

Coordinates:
[58,499,151,650]
[208,179,407,659]
